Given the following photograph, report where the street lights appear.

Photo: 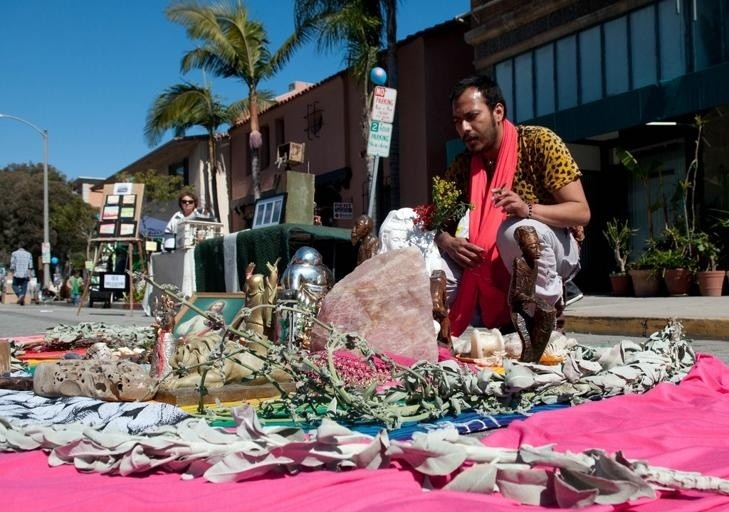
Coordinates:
[0,113,50,289]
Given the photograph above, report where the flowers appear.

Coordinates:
[413,176,474,233]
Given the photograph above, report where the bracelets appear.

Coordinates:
[525,200,533,219]
[433,230,448,242]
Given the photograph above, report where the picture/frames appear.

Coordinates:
[249,193,288,230]
[99,182,138,238]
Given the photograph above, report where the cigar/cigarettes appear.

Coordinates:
[494,182,507,196]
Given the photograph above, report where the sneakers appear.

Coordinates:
[17,295,25,305]
[565,280,583,307]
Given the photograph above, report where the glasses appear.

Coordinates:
[181,199,195,204]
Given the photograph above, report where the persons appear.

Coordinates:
[150,294,177,380]
[379,205,424,255]
[238,255,282,342]
[160,191,200,253]
[175,297,228,338]
[433,70,592,336]
[350,213,380,265]
[65,268,84,303]
[9,240,34,305]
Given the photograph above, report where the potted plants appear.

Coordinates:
[628,221,724,296]
[603,217,632,296]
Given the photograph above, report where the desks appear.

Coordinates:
[145,218,368,308]
